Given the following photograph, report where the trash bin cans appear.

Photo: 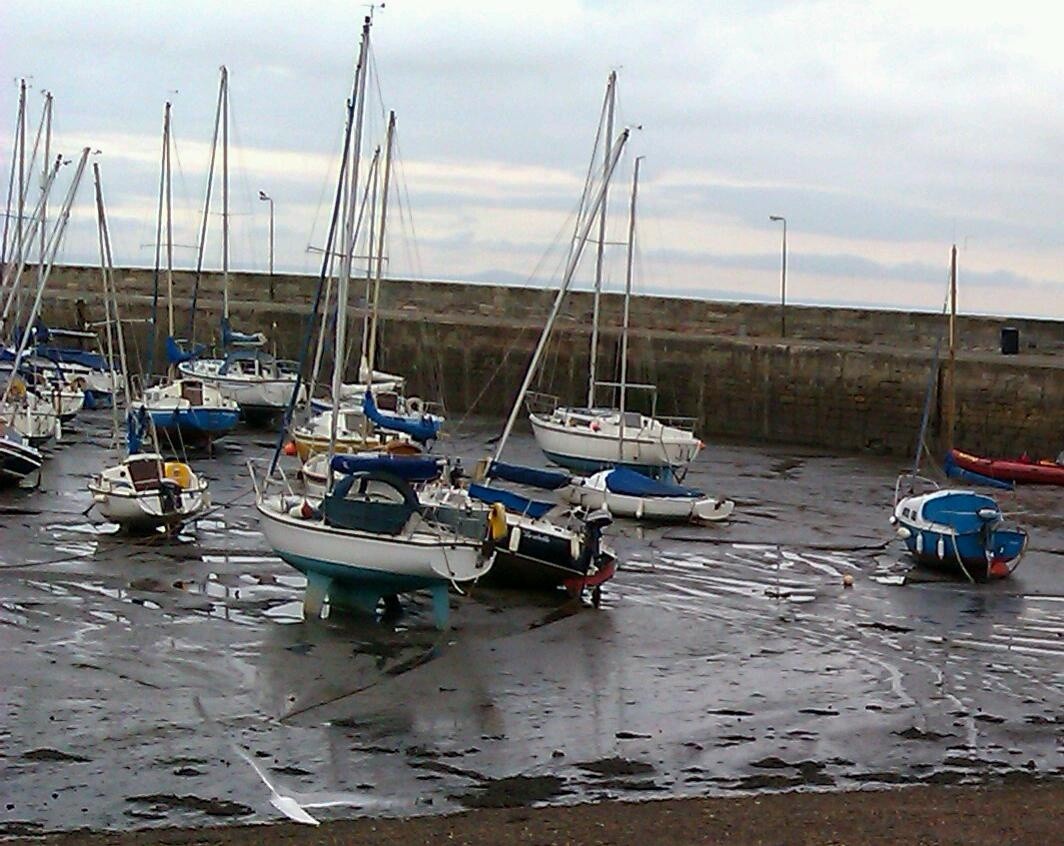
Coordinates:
[1001,328,1020,355]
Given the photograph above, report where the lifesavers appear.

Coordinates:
[406,396,423,415]
[165,462,189,487]
[492,502,507,540]
[13,381,26,395]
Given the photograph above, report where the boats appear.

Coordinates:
[888,473,1031,585]
[946,446,1064,485]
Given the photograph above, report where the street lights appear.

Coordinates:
[770,214,790,339]
[257,195,278,298]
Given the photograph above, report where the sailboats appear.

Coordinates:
[1,10,740,628]
